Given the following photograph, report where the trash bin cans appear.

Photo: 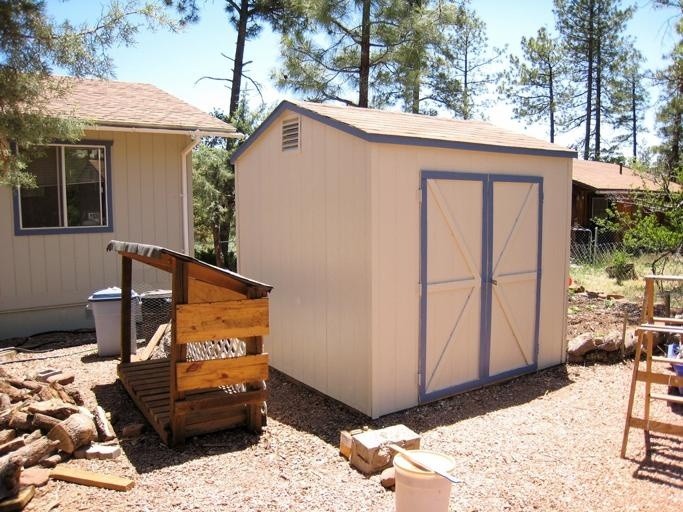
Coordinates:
[85,287,140,356]
[141,290,172,346]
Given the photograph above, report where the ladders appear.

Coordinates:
[621,274,682,461]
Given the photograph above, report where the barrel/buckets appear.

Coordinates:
[393,450,456,512]
